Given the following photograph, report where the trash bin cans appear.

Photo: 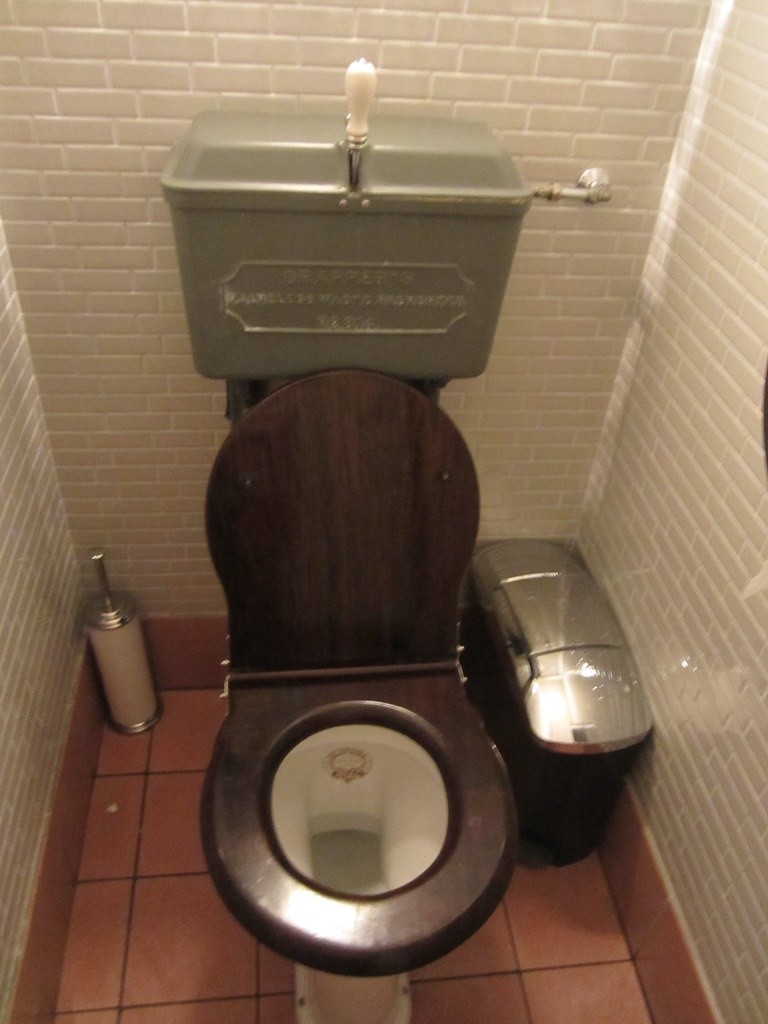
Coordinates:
[462,539,656,863]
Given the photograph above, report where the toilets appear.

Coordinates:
[164,139,536,976]
